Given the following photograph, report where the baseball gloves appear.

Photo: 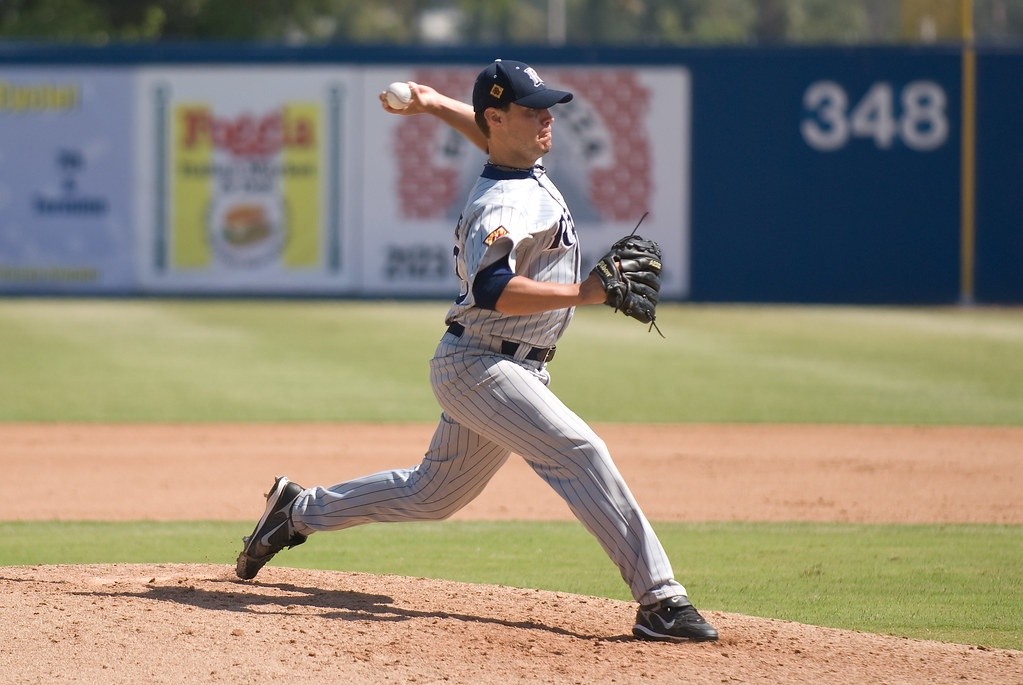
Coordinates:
[589,233,663,325]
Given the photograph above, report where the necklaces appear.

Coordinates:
[486,161,546,174]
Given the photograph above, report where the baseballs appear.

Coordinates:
[386,82,411,110]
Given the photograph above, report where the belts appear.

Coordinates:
[447,321,556,363]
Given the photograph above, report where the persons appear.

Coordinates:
[236,60,719,643]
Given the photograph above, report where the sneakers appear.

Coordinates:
[234,475,308,580]
[631,595,718,642]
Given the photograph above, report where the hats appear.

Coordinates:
[472,58,573,113]
